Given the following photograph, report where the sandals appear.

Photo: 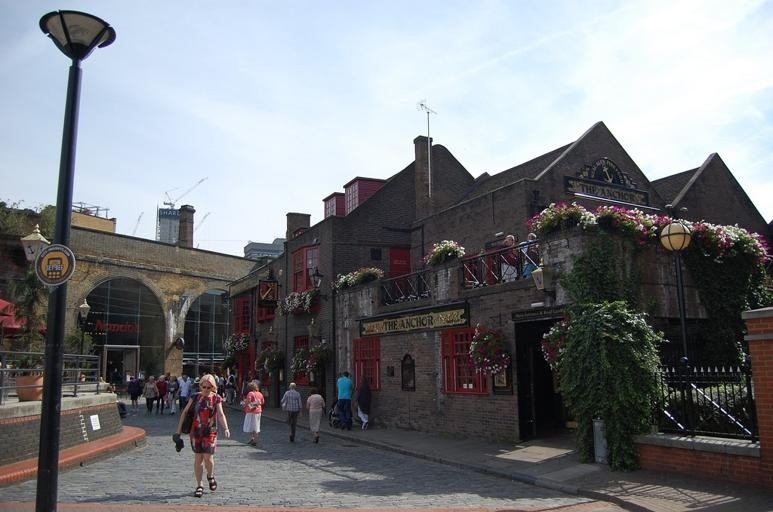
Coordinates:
[195,487,204,497]
[207,475,217,490]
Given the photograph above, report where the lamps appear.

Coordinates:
[308,265,324,288]
[308,317,321,337]
[530,258,554,291]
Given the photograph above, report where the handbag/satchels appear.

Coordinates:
[249,399,261,409]
[281,401,287,412]
[180,403,195,434]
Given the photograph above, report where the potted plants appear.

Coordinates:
[0,199,56,401]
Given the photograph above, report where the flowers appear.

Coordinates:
[289,343,330,374]
[527,202,769,266]
[331,268,385,293]
[277,289,319,320]
[539,319,570,371]
[468,322,513,376]
[423,240,467,267]
[225,334,250,352]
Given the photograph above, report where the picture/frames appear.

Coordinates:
[491,363,513,395]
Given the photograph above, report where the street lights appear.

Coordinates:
[657,220,697,438]
[71,295,91,394]
[33,5,117,511]
[20,222,53,377]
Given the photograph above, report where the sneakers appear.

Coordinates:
[361,422,368,430]
[248,438,255,444]
[290,434,293,441]
[316,435,319,443]
[252,442,257,445]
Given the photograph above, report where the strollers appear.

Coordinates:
[329,397,341,429]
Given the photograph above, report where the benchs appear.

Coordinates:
[107,384,129,400]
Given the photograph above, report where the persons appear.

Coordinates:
[336,372,353,431]
[280,382,303,442]
[306,387,326,443]
[523,233,541,278]
[501,235,520,282]
[355,375,371,431]
[241,383,265,446]
[177,373,231,497]
[110,366,239,416]
